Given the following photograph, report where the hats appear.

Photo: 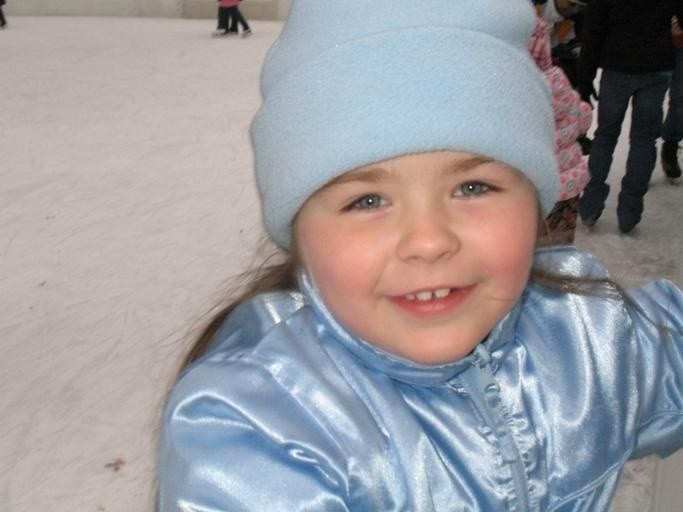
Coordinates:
[250,0,560,251]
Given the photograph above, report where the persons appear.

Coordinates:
[154,0,683,512]
[527,0,683,245]
[211,0,252,39]
